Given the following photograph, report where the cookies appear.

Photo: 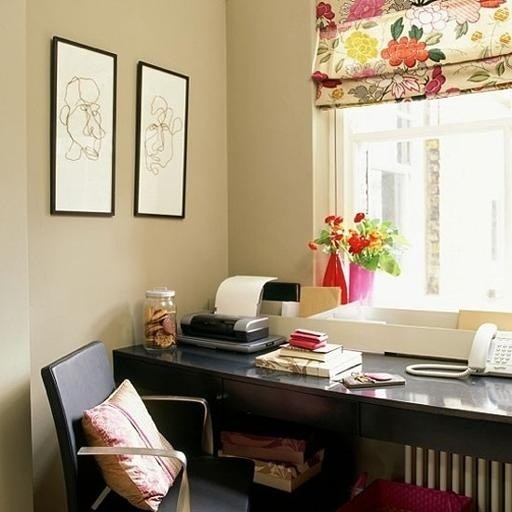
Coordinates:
[145,305,176,348]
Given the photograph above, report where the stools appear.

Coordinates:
[331,480,475,511]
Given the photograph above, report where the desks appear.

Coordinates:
[111,332,512,511]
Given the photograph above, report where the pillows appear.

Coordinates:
[82,378,184,512]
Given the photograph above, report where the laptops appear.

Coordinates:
[176,335,287,353]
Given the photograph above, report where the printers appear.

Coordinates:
[180,287,270,343]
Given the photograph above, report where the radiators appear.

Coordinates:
[403,444,511,511]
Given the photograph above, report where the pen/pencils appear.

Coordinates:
[352,373,362,383]
[364,376,375,384]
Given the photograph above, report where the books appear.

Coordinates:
[252,343,366,380]
[341,372,406,387]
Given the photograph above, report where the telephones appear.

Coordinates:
[405,322,512,378]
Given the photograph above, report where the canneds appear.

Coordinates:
[144,289,177,352]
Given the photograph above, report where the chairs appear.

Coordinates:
[40,340,255,512]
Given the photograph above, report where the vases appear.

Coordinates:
[349,263,373,305]
[322,250,348,304]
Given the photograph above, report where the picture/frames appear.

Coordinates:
[132,59,190,219]
[48,36,115,219]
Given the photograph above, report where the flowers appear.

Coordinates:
[347,213,410,276]
[311,1,512,108]
[307,216,352,253]
